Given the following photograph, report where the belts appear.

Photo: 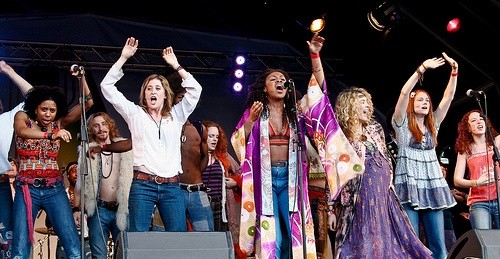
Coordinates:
[133,171,178,185]
[181,185,207,191]
[16,175,63,188]
[97,199,119,211]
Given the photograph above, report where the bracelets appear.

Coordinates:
[49,133,53,140]
[175,66,183,72]
[311,53,320,58]
[422,63,428,70]
[44,133,49,139]
[416,68,423,81]
[451,72,458,76]
[470,180,479,188]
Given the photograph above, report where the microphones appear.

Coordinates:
[70,65,84,75]
[280,79,293,89]
[466,89,484,96]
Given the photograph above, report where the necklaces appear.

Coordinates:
[147,109,164,139]
[182,125,186,142]
[350,128,384,168]
[100,152,113,179]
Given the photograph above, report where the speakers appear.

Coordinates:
[446,229,499,258]
[112,231,235,259]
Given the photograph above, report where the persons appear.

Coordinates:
[334,52,500,259]
[232,33,367,259]
[0,37,242,259]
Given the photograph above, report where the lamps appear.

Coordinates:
[435,7,466,36]
[295,7,329,34]
[225,53,249,97]
[366,0,402,41]
